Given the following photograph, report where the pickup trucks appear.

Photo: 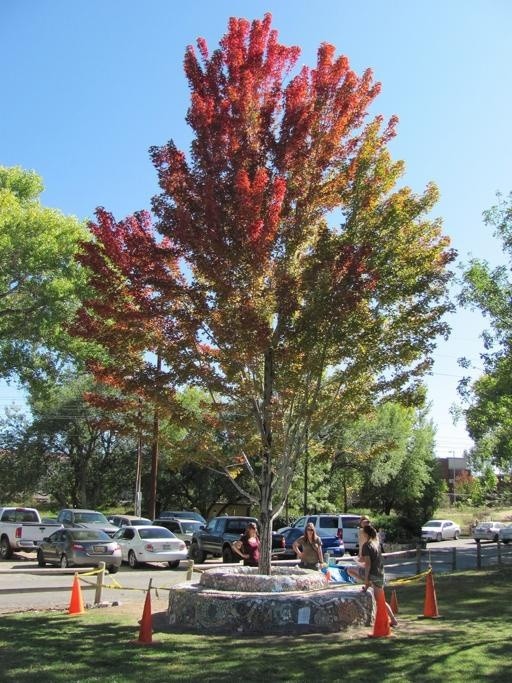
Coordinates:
[1,506,65,557]
[190,515,286,567]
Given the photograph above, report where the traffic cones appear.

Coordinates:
[416,570,443,619]
[67,571,84,614]
[388,589,399,613]
[130,591,160,646]
[369,586,397,638]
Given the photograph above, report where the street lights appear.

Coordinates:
[448,449,456,502]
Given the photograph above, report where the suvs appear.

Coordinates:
[154,516,206,550]
[290,512,369,556]
[58,508,121,538]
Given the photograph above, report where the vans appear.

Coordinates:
[159,509,206,525]
[107,513,153,532]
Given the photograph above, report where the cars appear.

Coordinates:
[420,519,461,541]
[471,521,505,543]
[35,527,123,574]
[498,523,512,543]
[278,527,346,559]
[108,524,189,570]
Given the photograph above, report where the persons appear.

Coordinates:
[232,523,260,567]
[293,523,323,571]
[346,516,397,626]
